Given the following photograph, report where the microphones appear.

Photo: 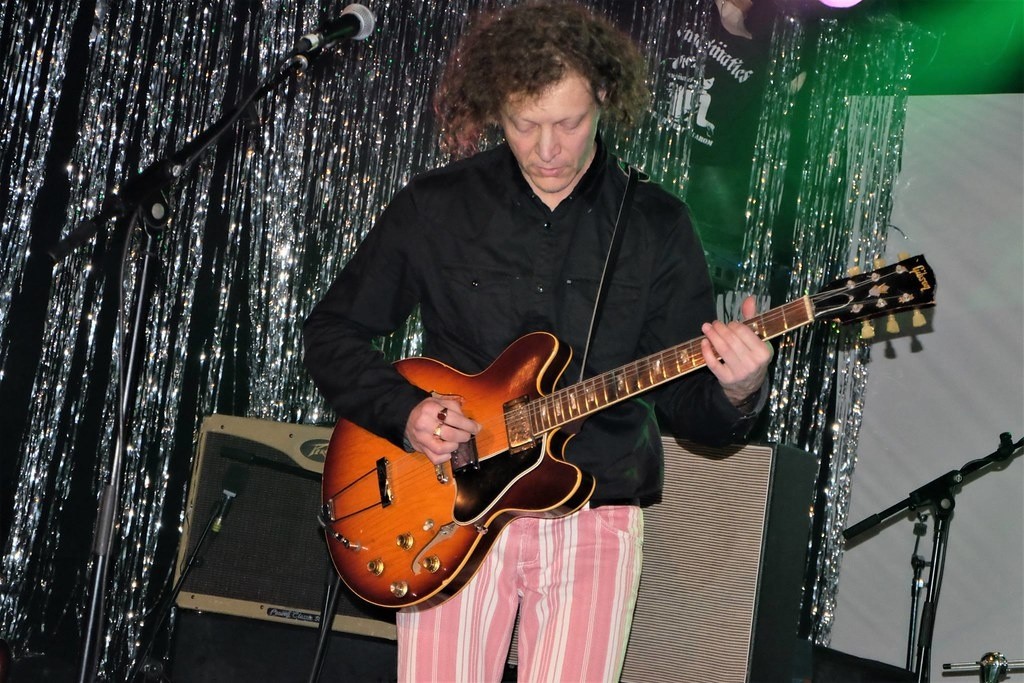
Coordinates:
[295,4,375,52]
[207,486,236,541]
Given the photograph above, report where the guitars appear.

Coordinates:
[322,251,939,615]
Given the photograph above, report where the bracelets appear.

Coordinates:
[736,388,760,408]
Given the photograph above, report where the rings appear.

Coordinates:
[432,424,443,439]
[437,408,449,424]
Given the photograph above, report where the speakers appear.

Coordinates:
[172,413,401,641]
[508,434,817,683]
[171,614,397,683]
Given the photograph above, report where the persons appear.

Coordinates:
[302,1,775,683]
